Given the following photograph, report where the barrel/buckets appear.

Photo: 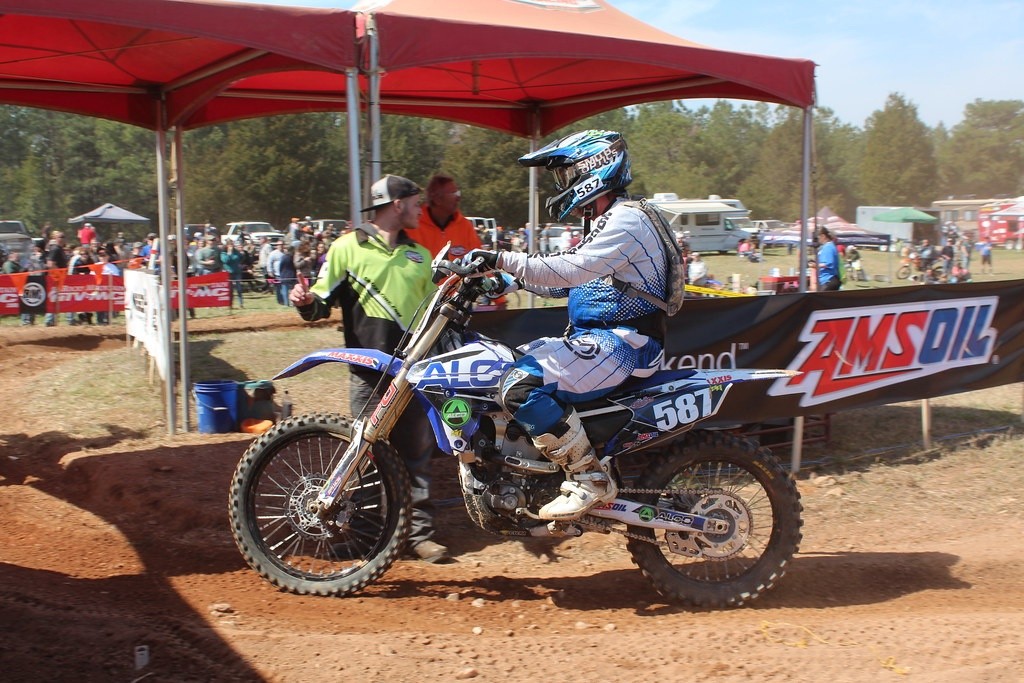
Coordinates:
[193,379,239,434]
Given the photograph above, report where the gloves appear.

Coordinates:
[460,248,500,270]
[486,274,525,300]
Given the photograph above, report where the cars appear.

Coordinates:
[750,219,785,231]
[184,223,217,243]
[0,220,35,253]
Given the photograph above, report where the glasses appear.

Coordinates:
[97,251,107,257]
[446,188,462,197]
[551,167,572,191]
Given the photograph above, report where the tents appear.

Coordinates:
[67,202,151,233]
[760,207,891,282]
[990,203,1023,236]
[0,0,815,472]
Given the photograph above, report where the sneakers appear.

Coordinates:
[408,539,450,563]
[328,538,373,560]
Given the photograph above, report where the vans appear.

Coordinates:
[282,219,348,238]
[536,225,584,254]
[463,217,498,249]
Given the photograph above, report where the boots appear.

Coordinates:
[531,405,619,520]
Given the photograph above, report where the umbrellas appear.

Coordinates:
[872,207,939,223]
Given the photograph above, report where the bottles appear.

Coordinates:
[282,390,292,416]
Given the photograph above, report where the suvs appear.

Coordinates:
[220,223,286,247]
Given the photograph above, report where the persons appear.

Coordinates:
[980,237,992,274]
[838,245,860,268]
[405,174,505,305]
[473,222,581,252]
[808,226,840,290]
[285,172,448,561]
[459,129,681,521]
[918,221,972,284]
[737,240,762,263]
[676,234,707,287]
[0,216,353,325]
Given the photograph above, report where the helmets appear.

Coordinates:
[518,128,633,224]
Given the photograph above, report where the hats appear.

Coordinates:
[206,235,217,240]
[226,238,233,244]
[96,246,108,253]
[360,174,422,212]
[168,235,176,240]
[692,252,699,256]
[292,218,299,220]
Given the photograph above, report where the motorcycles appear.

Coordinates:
[227,241,804,612]
[898,250,940,280]
